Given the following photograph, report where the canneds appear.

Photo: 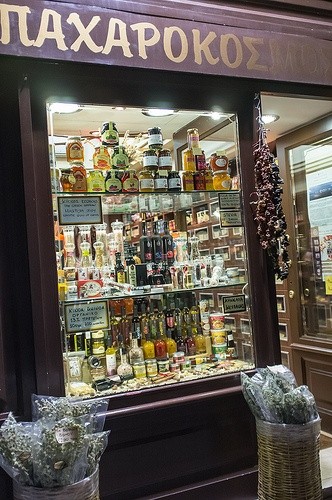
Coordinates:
[60,122,234,191]
[132,351,192,379]
[91,332,105,357]
[64,267,76,281]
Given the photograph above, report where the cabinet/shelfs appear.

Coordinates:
[45,98,256,403]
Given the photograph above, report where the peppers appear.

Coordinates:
[251,147,291,281]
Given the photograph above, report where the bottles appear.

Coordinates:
[61,220,227,302]
[64,305,206,380]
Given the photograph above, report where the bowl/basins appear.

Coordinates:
[210,312,230,358]
